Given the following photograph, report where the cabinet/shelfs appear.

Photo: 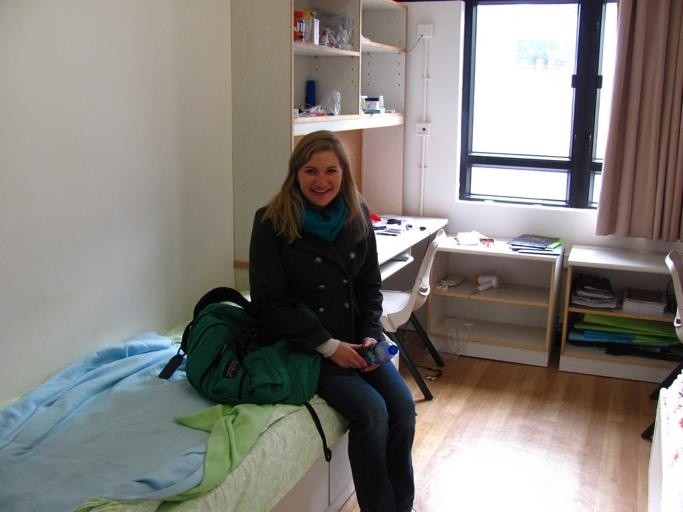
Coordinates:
[230,1,408,294]
[559,244,679,381]
[425,233,565,367]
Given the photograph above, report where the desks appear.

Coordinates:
[369,214,448,288]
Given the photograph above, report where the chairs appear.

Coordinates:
[641,245,682,445]
[382,227,447,401]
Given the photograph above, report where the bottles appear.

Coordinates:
[365,97,380,114]
[354,342,396,367]
[295,11,305,41]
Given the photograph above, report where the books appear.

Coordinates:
[572,275,617,310]
[621,288,666,317]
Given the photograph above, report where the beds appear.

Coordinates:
[1,321,400,507]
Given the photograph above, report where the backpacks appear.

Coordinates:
[159,287,321,406]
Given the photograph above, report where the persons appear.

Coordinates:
[251,130,417,511]
